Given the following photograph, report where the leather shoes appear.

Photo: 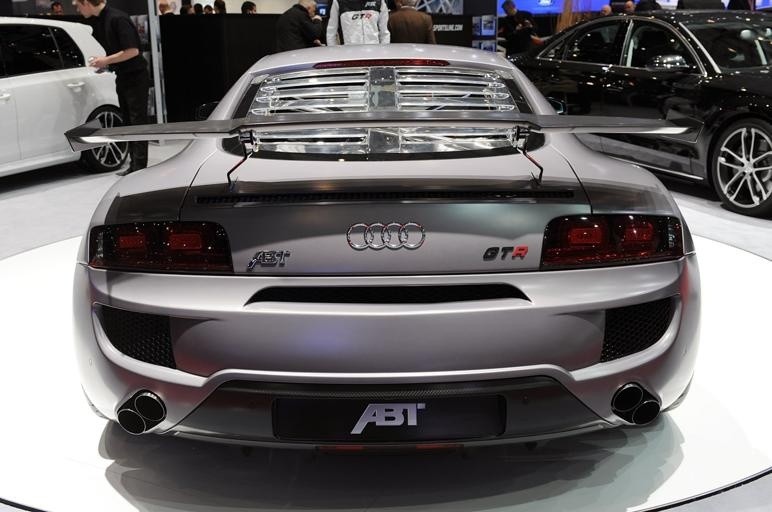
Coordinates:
[115,167,134,176]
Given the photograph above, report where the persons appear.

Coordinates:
[76,0,149,176]
[624,1,637,14]
[159,0,435,122]
[599,4,612,17]
[499,1,535,55]
[51,2,64,16]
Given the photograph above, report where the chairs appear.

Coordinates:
[575,32,609,63]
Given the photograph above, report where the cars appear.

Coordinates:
[507,9,772,218]
[60,40,704,448]
[0,14,130,180]
